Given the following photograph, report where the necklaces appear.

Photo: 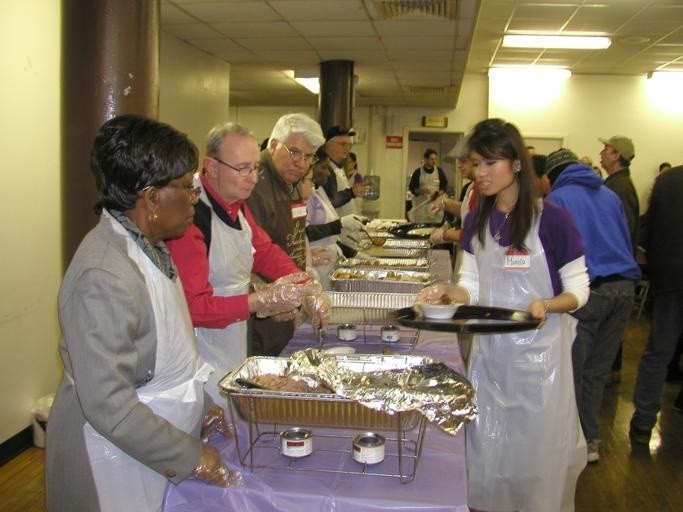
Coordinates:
[493,212,510,243]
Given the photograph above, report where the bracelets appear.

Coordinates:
[442,229,449,241]
[442,196,448,208]
[544,299,552,313]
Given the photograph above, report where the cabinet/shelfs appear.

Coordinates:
[319,291,422,357]
[216,355,434,486]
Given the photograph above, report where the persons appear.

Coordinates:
[626,164,683,450]
[343,152,364,218]
[293,166,333,268]
[596,134,641,387]
[665,333,683,411]
[42,112,241,511]
[580,157,592,168]
[428,134,481,278]
[658,163,674,173]
[530,154,553,197]
[164,120,335,415]
[245,112,329,357]
[324,125,373,258]
[408,148,450,228]
[311,150,368,288]
[412,117,593,512]
[525,145,535,157]
[542,147,643,468]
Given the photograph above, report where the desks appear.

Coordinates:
[163,248,469,512]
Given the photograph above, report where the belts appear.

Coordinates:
[591,274,626,288]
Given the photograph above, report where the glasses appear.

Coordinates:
[213,157,265,177]
[335,142,354,149]
[282,143,319,165]
[142,185,202,204]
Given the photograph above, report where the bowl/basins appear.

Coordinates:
[418,301,464,320]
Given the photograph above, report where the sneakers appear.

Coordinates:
[629,427,650,445]
[586,438,601,462]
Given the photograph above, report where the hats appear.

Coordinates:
[447,134,472,158]
[327,126,357,142]
[598,136,634,162]
[545,148,580,174]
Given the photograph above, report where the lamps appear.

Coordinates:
[486,67,683,81]
[501,31,612,50]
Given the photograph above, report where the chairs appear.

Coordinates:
[633,246,649,320]
